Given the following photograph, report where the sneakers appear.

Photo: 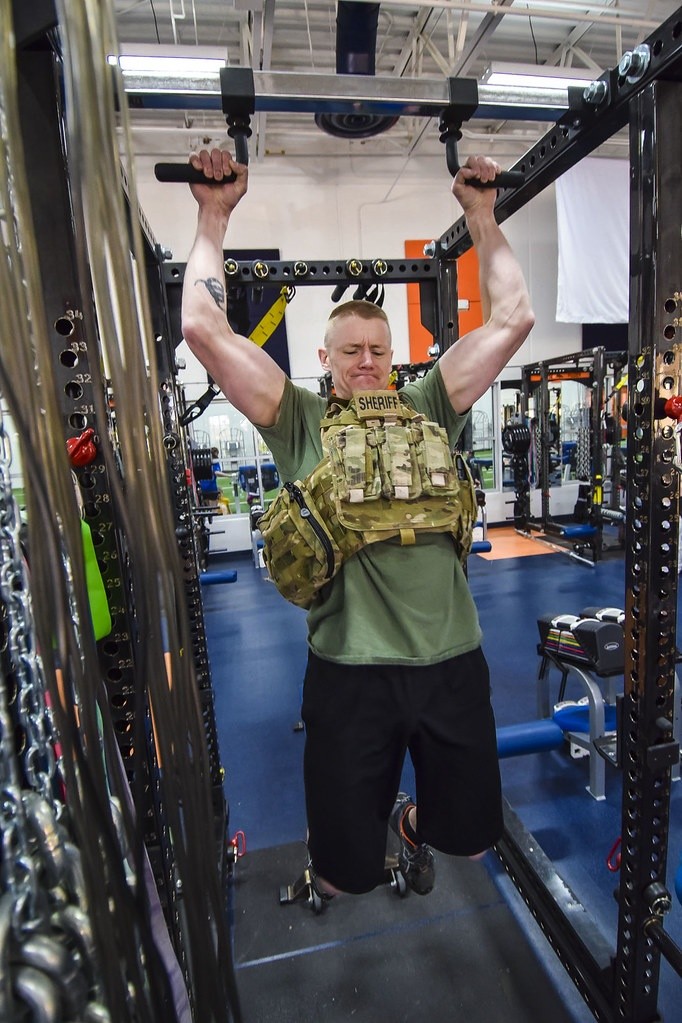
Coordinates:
[306,824,334,902]
[389,791,435,894]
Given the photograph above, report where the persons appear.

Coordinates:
[177,148,538,901]
